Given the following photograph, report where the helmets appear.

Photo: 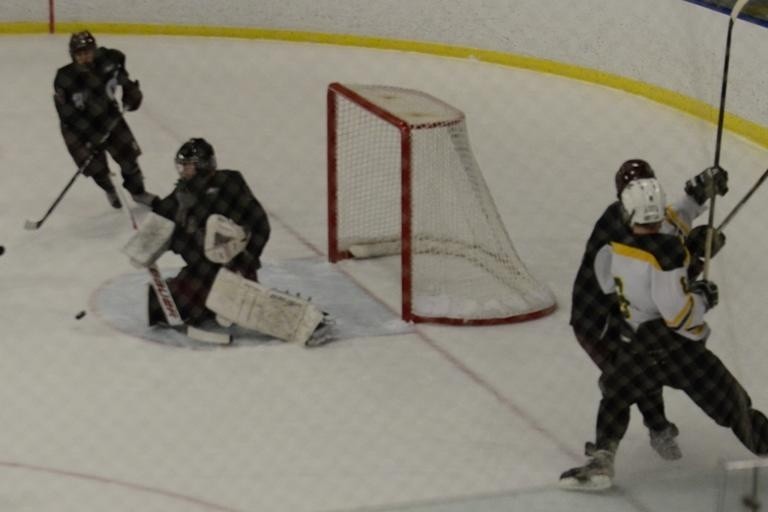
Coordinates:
[69,30,99,71]
[612,159,667,228]
[173,138,217,188]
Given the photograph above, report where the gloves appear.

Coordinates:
[691,278,719,312]
[687,224,726,267]
[121,86,144,112]
[682,165,728,207]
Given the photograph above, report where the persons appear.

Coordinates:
[51,30,162,208]
[557,176,768,494]
[569,158,729,463]
[144,137,341,347]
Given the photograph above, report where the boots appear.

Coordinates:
[557,442,615,485]
[131,191,161,207]
[649,419,684,462]
[104,189,123,210]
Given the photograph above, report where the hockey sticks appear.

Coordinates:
[703,0,748,281]
[25,80,139,230]
[107,172,232,343]
